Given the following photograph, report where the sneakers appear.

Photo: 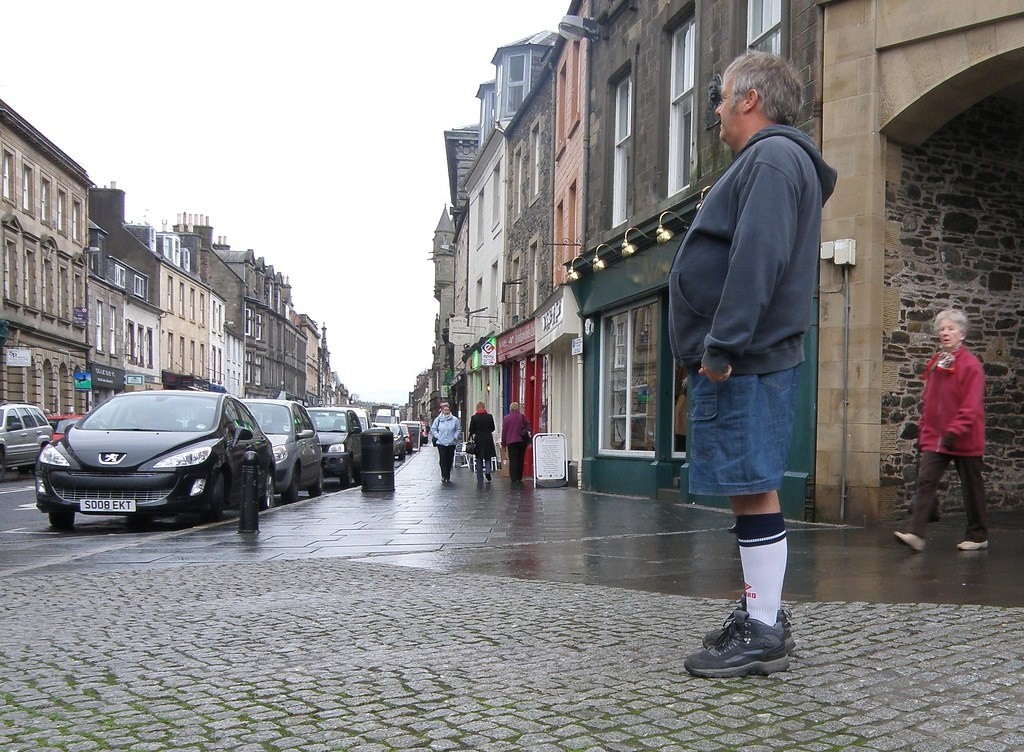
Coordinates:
[702,594,797,651]
[684,610,790,677]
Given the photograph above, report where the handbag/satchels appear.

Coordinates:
[431,435,438,447]
[520,414,531,441]
[466,432,475,455]
[908,492,941,523]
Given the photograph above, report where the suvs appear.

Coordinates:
[0,401,54,482]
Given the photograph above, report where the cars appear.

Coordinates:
[354,408,428,459]
[32,388,276,531]
[47,414,83,442]
[240,398,324,504]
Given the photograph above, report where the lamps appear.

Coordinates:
[592,243,622,273]
[655,210,689,245]
[621,227,656,257]
[566,255,593,284]
[697,185,711,209]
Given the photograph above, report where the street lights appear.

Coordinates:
[83,245,100,411]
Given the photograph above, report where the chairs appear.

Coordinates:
[196,407,215,428]
[453,432,497,472]
[334,419,345,430]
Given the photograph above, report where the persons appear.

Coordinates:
[430,403,461,482]
[669,50,837,678]
[501,401,529,483]
[893,309,988,551]
[470,401,497,482]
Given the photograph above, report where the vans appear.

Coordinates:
[306,407,362,486]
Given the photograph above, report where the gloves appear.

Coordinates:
[940,430,956,452]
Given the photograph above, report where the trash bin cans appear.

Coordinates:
[358,428,395,493]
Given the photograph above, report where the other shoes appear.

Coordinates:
[511,480,517,484]
[894,531,924,552]
[446,478,449,482]
[478,480,483,483]
[485,473,491,481]
[441,477,446,482]
[957,541,988,550]
[518,480,524,484]
